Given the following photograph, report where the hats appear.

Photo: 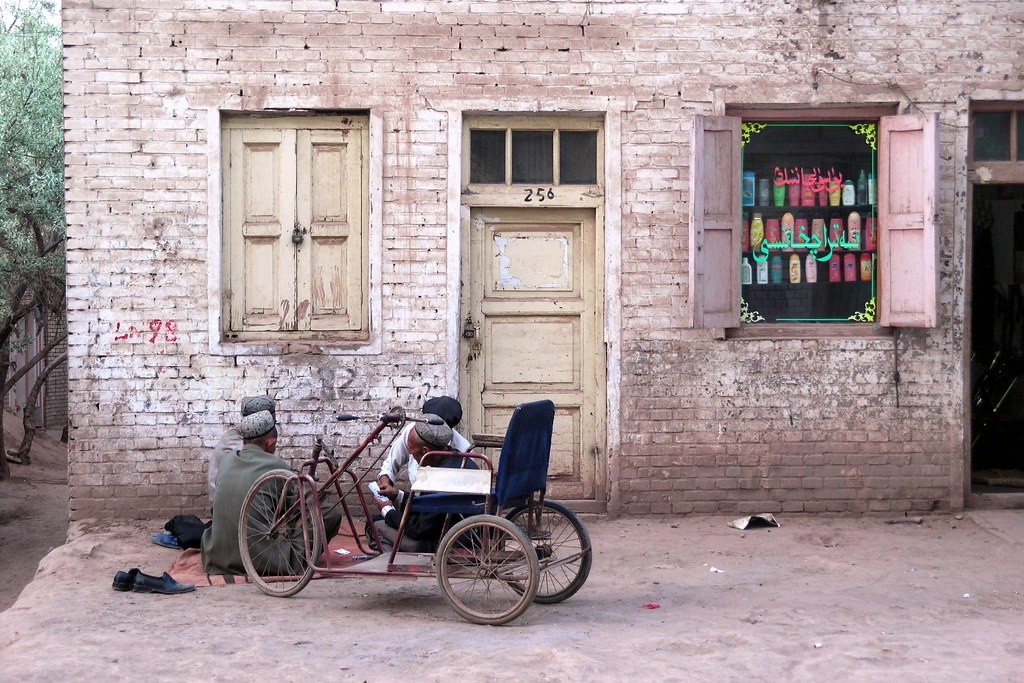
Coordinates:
[416,412,454,447]
[422,395,463,429]
[241,410,275,439]
[240,394,276,418]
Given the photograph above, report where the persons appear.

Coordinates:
[200,410,342,575]
[365,396,475,537]
[208,395,278,519]
[368,414,480,552]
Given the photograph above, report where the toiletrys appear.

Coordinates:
[743,170,877,207]
[742,211,878,252]
[742,253,872,285]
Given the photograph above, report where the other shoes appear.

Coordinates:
[152,532,180,549]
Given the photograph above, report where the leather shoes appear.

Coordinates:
[132,570,197,595]
[112,567,142,591]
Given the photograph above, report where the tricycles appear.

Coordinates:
[239,400,594,624]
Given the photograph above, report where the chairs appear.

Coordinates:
[406,397,556,513]
